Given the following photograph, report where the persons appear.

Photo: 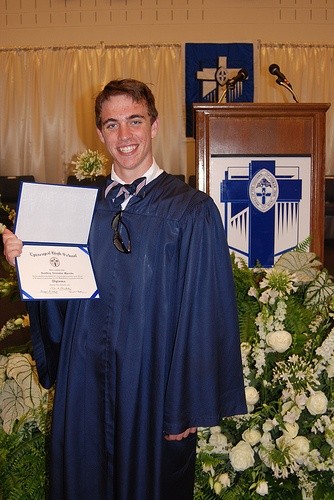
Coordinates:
[2,79,249,499]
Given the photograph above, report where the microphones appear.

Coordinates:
[228,69,249,86]
[269,64,292,90]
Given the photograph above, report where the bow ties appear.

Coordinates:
[104,177,147,208]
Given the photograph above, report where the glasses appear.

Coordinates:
[112,210,133,254]
